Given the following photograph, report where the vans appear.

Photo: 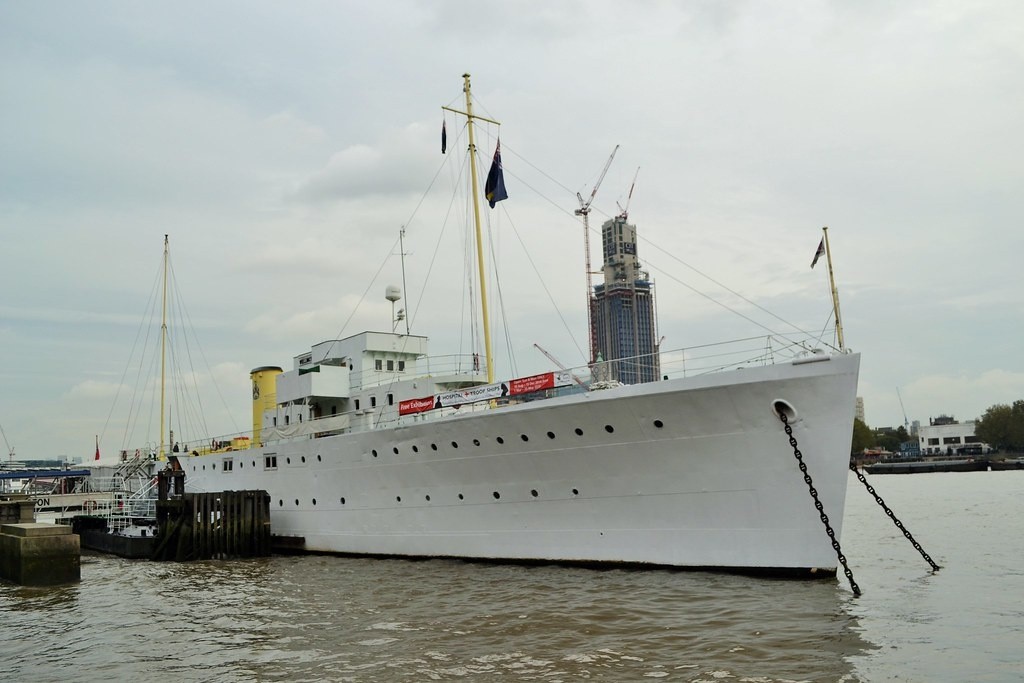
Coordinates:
[964,443,993,454]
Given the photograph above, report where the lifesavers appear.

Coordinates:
[153,476,158,485]
[135,448,139,459]
[84,500,97,510]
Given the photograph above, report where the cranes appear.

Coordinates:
[0,425,16,461]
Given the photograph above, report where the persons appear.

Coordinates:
[173,441,180,452]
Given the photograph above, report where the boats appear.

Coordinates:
[27,475,141,527]
[149,72,863,583]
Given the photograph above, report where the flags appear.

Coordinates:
[810,236,825,269]
[484,138,508,208]
